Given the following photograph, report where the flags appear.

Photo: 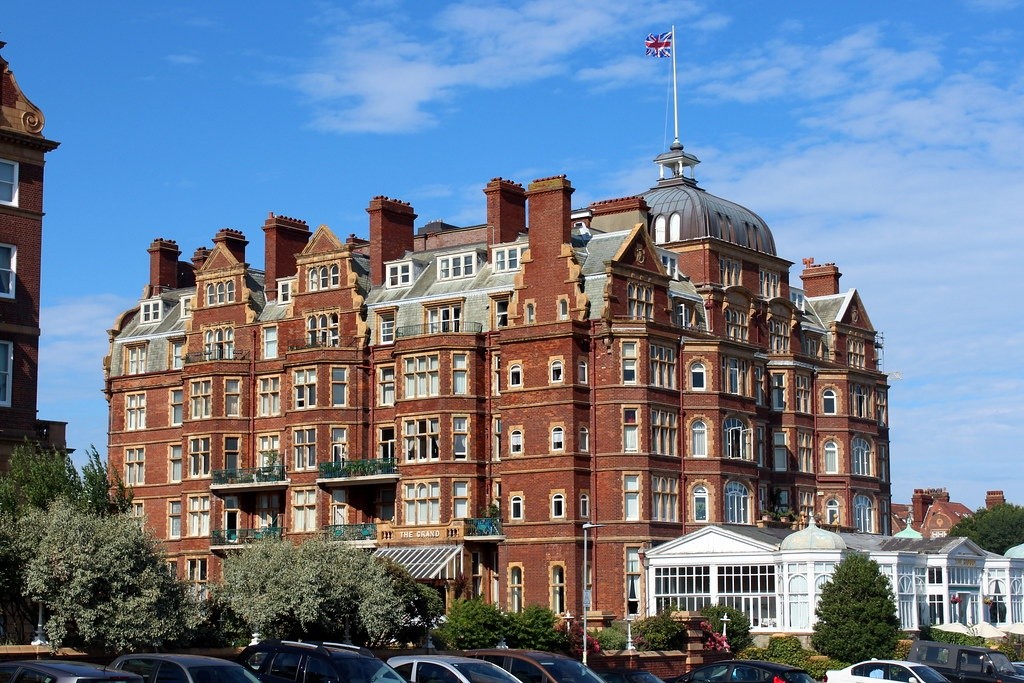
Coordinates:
[645,31,672,57]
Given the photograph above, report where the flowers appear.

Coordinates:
[984,598,992,608]
[952,595,963,602]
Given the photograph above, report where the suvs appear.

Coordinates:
[231,638,410,683]
[903,640,1024,683]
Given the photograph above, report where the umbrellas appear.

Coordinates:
[970,622,1006,637]
[997,623,1024,634]
[931,623,972,636]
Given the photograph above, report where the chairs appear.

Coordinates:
[744,669,757,681]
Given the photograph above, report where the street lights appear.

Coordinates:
[581,520,608,676]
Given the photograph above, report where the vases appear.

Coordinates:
[951,600,957,605]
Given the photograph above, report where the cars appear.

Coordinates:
[0,658,145,683]
[371,653,523,683]
[108,653,260,683]
[574,666,667,683]
[821,658,952,683]
[663,658,817,683]
[997,661,1024,673]
[458,647,608,683]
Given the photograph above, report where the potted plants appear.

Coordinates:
[780,513,788,522]
[761,511,773,521]
